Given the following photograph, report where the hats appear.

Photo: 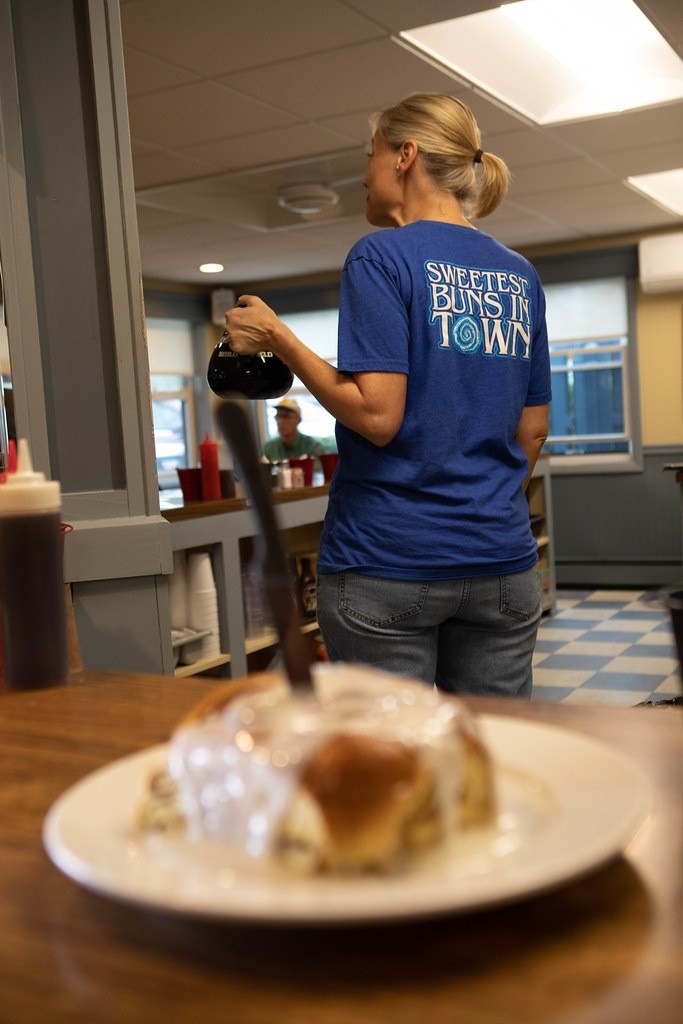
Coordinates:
[272,398,300,416]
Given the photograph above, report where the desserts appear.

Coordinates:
[148,662,498,867]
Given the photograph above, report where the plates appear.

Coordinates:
[43,714,653,924]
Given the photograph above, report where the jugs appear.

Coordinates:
[207,306,295,400]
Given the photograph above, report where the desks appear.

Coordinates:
[0,671,683,1024]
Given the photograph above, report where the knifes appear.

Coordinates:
[214,402,315,691]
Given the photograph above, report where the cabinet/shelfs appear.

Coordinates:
[160,449,557,680]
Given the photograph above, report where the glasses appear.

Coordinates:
[275,415,296,420]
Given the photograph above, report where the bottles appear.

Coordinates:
[0,438,68,690]
[267,457,293,492]
[298,558,317,618]
[200,432,221,501]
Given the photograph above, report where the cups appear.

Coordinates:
[289,456,311,487]
[318,454,337,481]
[176,466,199,499]
[168,551,221,669]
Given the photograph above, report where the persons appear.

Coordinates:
[260,398,324,474]
[207,90,552,702]
